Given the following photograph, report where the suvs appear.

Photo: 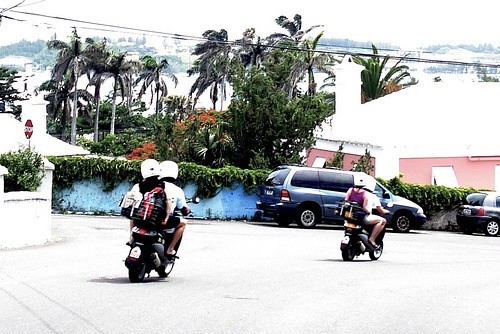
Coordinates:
[255,164,429,233]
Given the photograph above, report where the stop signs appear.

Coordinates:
[24,120,34,140]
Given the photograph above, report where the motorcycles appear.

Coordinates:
[125,195,201,282]
[335,191,392,261]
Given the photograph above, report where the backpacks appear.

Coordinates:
[134,180,167,232]
[118,191,145,219]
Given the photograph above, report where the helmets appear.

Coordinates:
[159,161,179,180]
[364,175,377,192]
[140,159,161,178]
[351,172,367,188]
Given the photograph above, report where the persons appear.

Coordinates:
[129,159,191,259]
[344,173,390,249]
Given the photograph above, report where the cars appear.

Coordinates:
[456,194,500,237]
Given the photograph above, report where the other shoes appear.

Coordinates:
[166,249,177,256]
[126,237,136,246]
[369,240,379,248]
